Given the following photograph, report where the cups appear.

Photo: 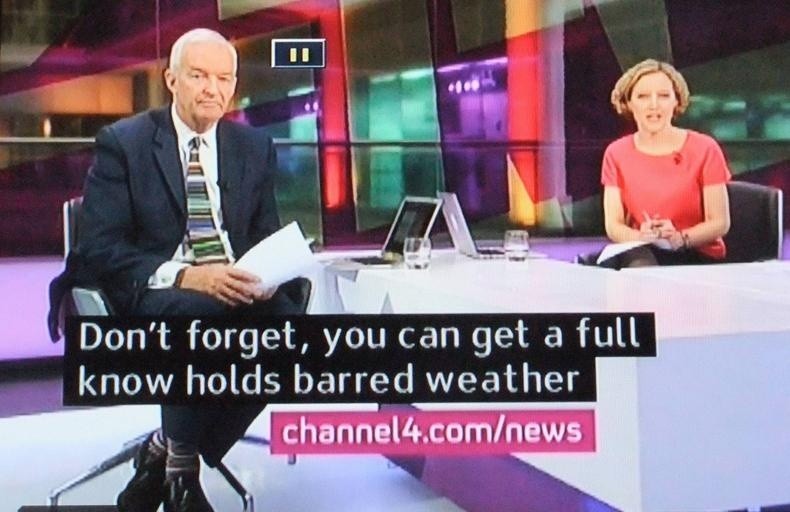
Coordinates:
[403,239,431,269]
[504,230,529,261]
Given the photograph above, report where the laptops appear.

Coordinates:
[318,195,445,270]
[436,191,547,259]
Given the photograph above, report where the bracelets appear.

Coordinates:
[680,231,691,250]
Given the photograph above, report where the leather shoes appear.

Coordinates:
[117,434,215,511]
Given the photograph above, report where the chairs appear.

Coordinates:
[723,180,784,261]
[50,196,314,512]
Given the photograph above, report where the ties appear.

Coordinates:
[180,139,227,265]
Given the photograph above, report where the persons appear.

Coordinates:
[64,27,313,511]
[600,59,733,271]
